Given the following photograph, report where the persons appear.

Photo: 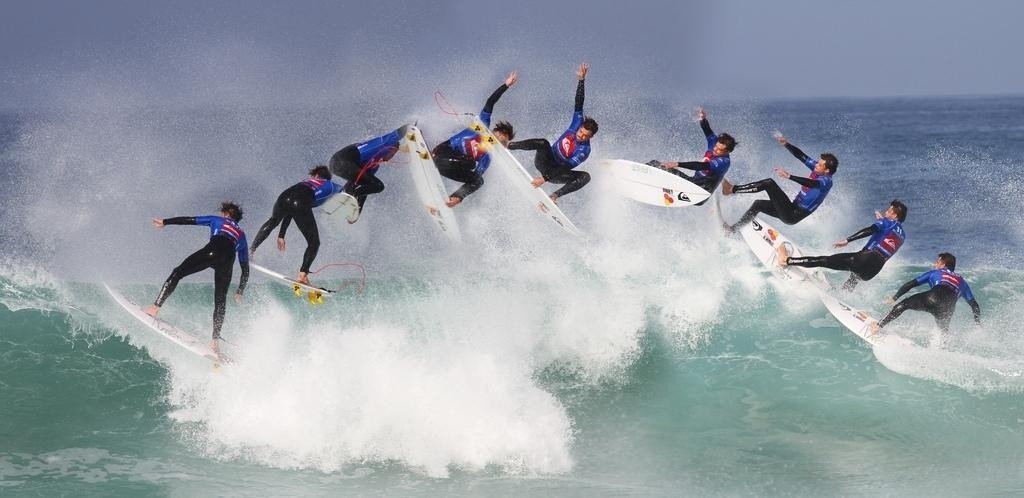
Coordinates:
[327,122,418,224]
[721,132,839,233]
[495,62,599,205]
[141,202,251,354]
[645,106,736,207]
[249,164,345,286]
[431,70,520,208]
[777,199,911,294]
[870,251,982,343]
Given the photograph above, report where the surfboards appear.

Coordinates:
[400,125,462,242]
[104,284,239,374]
[470,115,582,236]
[247,261,334,306]
[739,215,827,292]
[313,193,360,224]
[818,293,900,347]
[591,158,711,207]
